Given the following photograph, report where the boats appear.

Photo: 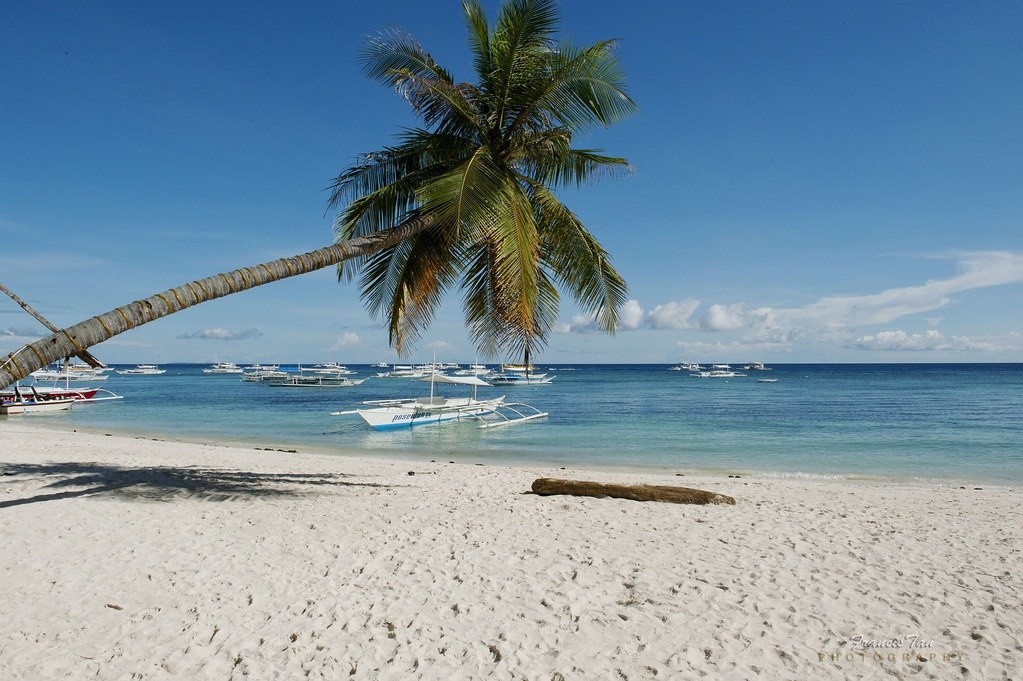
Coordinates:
[371,362,390,368]
[202,360,244,374]
[390,363,547,378]
[668,360,773,378]
[117,370,167,375]
[268,362,369,387]
[30,360,115,380]
[137,362,157,369]
[490,377,557,385]
[0,357,124,403]
[1,400,75,415]
[299,361,358,376]
[758,378,778,383]
[328,352,549,430]
[240,370,287,381]
[243,362,280,370]
